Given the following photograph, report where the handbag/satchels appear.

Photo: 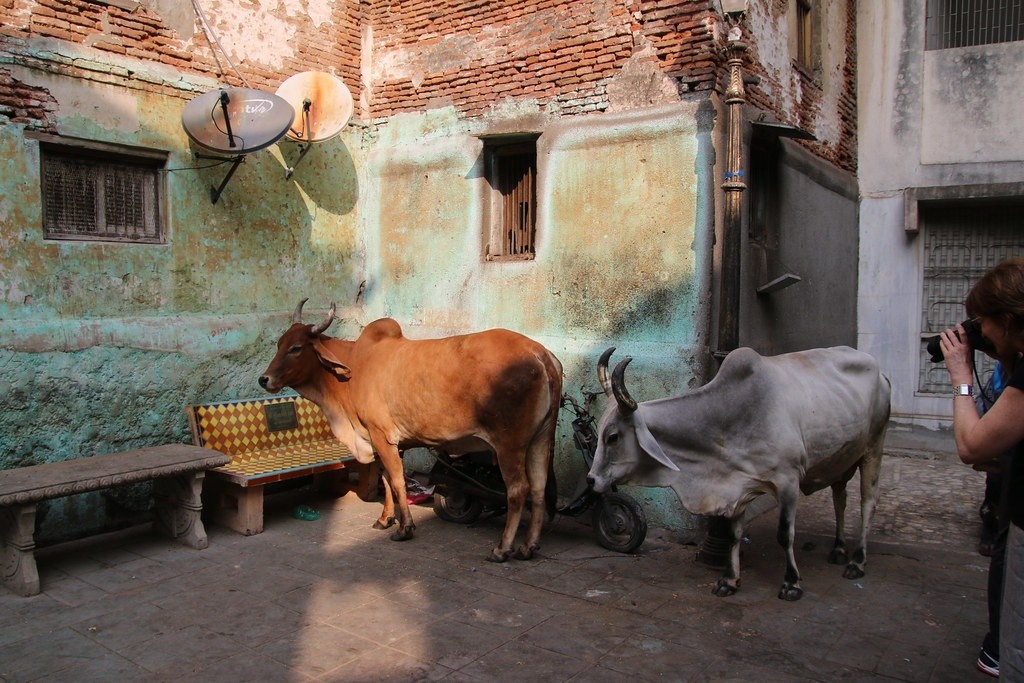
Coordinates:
[966,372,1013,474]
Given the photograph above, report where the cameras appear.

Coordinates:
[925,318,986,364]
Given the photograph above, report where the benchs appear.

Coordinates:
[185,393,404,536]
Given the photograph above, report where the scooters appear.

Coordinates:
[427,385,647,554]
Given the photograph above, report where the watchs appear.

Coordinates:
[953,383,973,400]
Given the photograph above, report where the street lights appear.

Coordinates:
[713,0,750,361]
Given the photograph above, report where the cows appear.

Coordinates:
[586,345,893,603]
[259,296,563,563]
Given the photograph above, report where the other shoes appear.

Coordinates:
[977,646,1000,677]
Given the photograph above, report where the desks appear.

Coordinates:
[0,443,232,597]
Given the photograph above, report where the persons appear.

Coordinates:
[939,257,1024,683]
